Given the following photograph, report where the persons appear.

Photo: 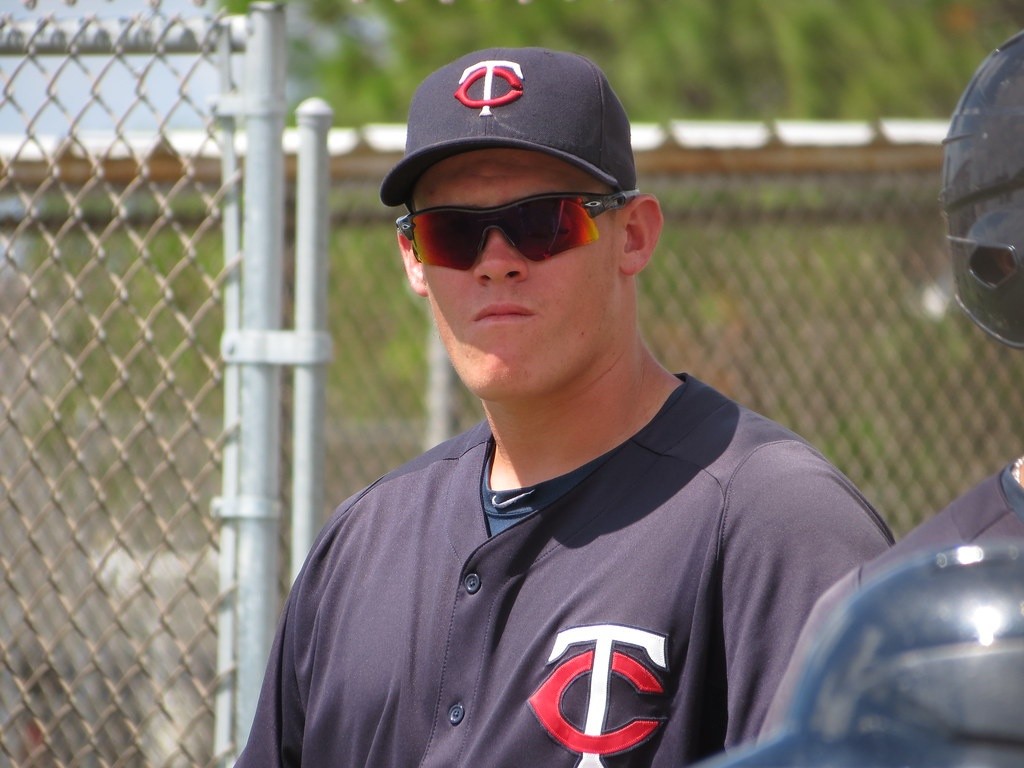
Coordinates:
[233,30,1024,768]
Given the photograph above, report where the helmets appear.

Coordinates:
[692,542,1024,768]
[939,27,1024,349]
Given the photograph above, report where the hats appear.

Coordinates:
[380,48,636,206]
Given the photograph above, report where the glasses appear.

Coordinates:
[396,188,640,271]
